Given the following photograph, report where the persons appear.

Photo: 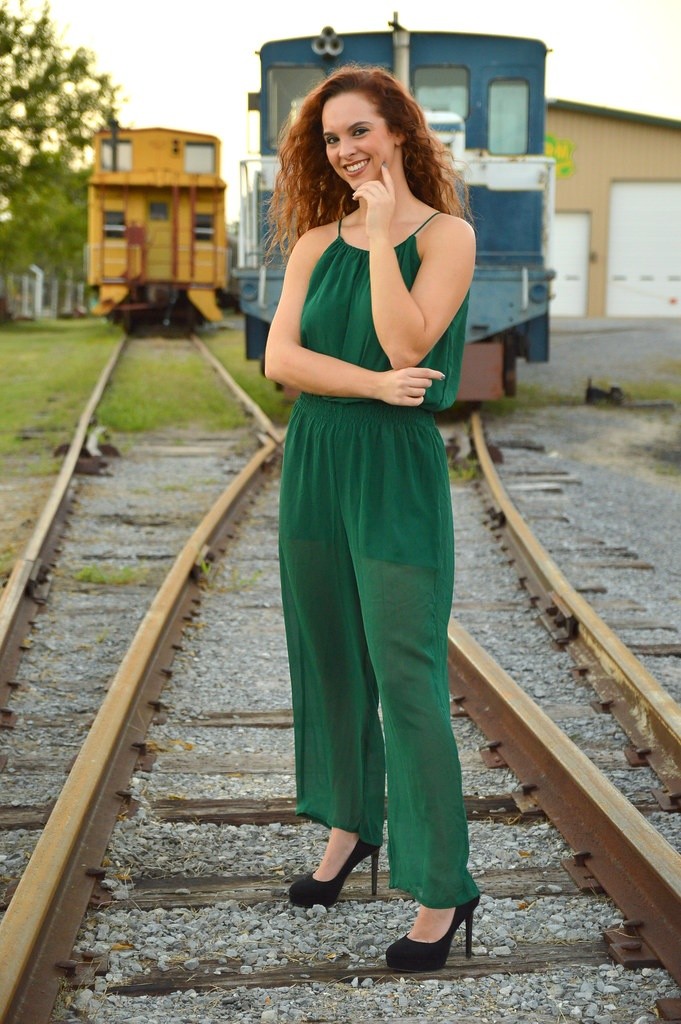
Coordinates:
[264,69,481,971]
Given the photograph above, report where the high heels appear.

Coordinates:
[289,838,381,908]
[386,896,480,973]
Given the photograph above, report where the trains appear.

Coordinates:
[230,10,557,419]
[87,126,228,335]
